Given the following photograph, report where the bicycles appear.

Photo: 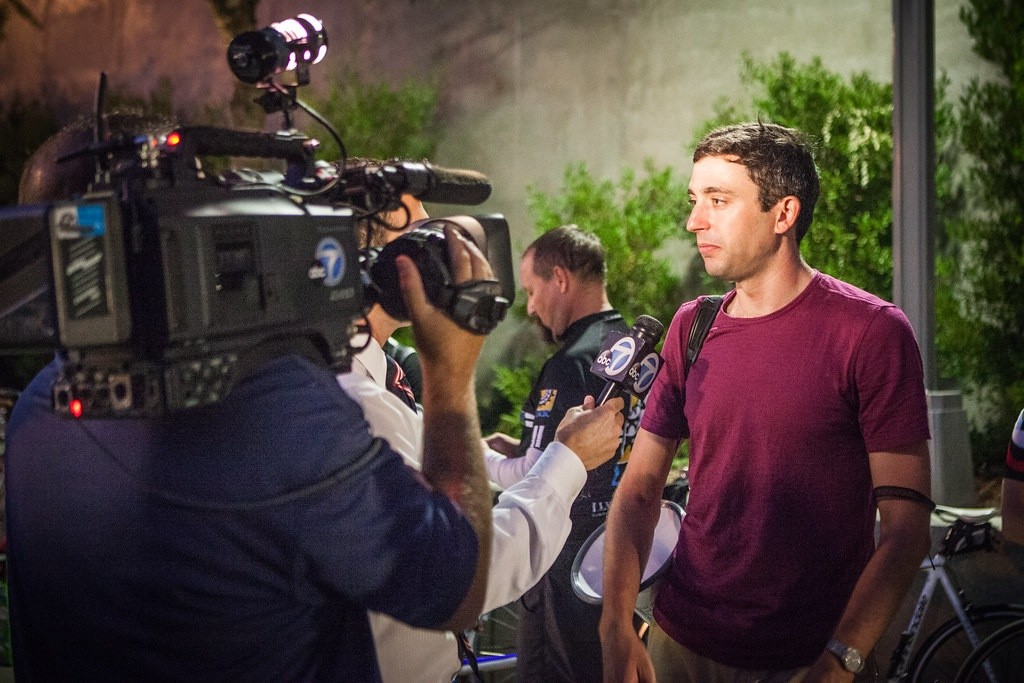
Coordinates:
[453,481,524,681]
[664,466,1023,683]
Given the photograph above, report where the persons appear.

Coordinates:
[331,156,625,683]
[479,223,662,683]
[599,121,931,683]
[1,107,497,683]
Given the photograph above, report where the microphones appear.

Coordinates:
[383,162,493,205]
[590,315,668,408]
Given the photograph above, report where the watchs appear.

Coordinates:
[827,639,866,673]
